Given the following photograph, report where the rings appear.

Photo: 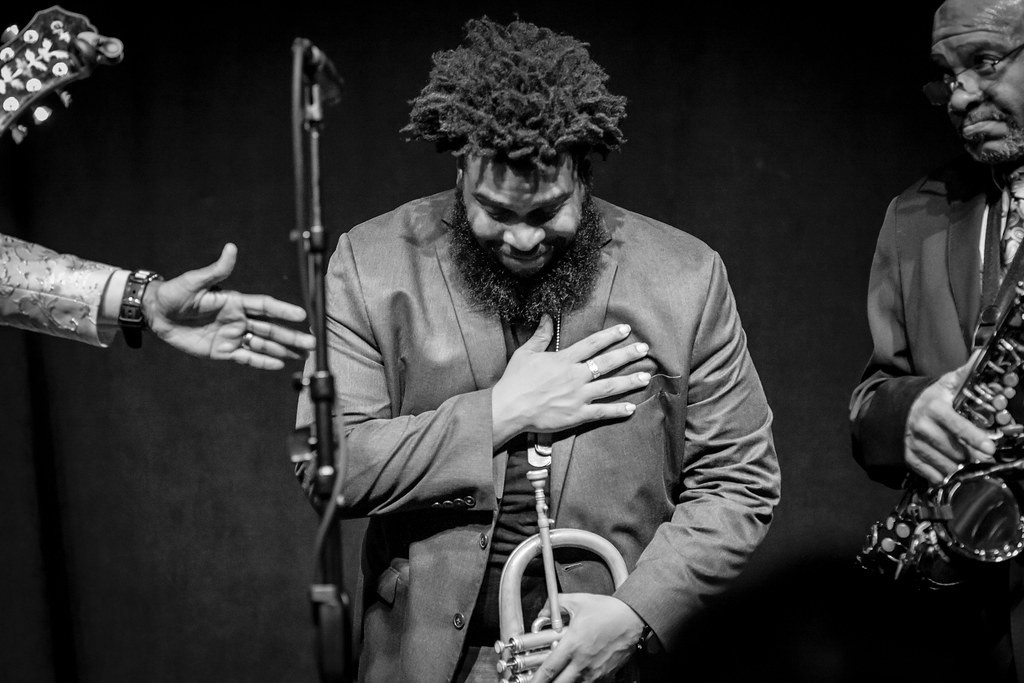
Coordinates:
[243,332,254,347]
[586,360,600,379]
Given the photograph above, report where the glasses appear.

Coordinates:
[922,43,1024,107]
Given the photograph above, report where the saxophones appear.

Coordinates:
[845,222,1024,612]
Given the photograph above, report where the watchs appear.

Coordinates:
[116,268,162,351]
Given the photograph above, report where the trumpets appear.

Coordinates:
[489,464,649,683]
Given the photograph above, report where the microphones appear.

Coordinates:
[297,40,344,90]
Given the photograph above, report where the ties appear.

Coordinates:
[998,169,1024,303]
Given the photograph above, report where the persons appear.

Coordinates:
[842,2,1024,492]
[0,232,320,374]
[283,19,785,683]
